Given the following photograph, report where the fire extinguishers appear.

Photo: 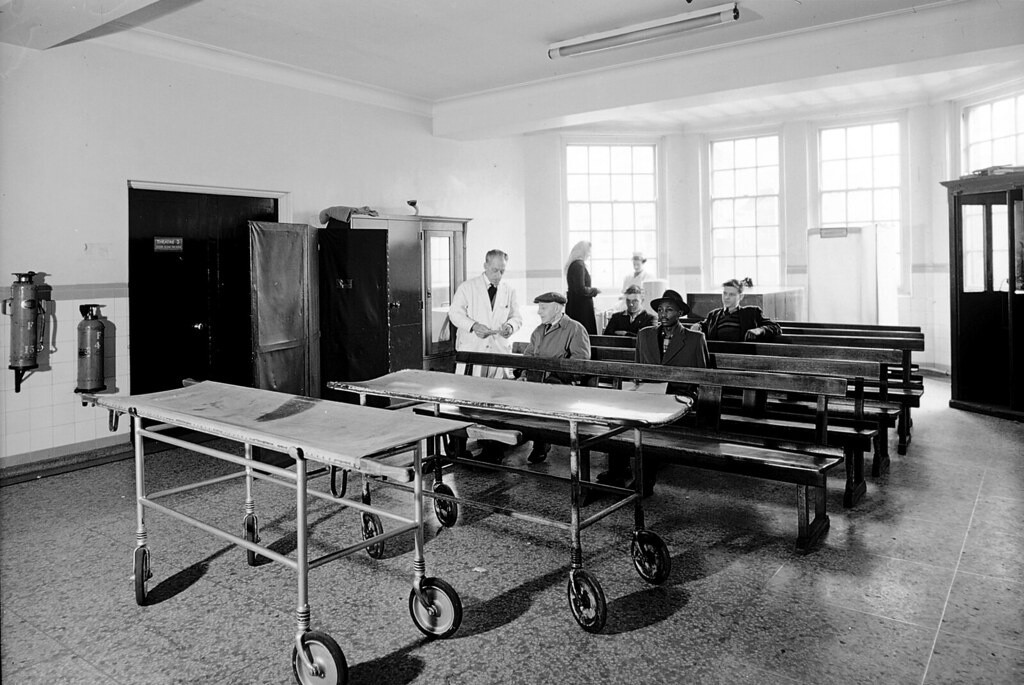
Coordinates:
[74,302,109,394]
[7,271,46,371]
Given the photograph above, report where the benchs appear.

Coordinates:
[784,333,929,451]
[779,327,925,390]
[772,322,920,376]
[589,335,903,478]
[450,350,847,546]
[510,342,879,503]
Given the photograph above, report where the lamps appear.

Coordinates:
[547,2,740,61]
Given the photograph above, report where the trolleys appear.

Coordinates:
[82,378,474,685]
[325,369,695,632]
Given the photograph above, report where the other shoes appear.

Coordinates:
[619,480,653,500]
[449,439,474,460]
[528,443,551,464]
[597,464,632,484]
[462,450,505,470]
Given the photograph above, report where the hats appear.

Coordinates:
[534,292,566,304]
[650,290,691,316]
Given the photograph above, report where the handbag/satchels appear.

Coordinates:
[319,206,379,224]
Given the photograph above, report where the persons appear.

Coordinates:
[691,280,781,343]
[604,286,657,339]
[517,292,591,388]
[619,254,653,302]
[637,290,711,369]
[565,239,600,335]
[449,249,522,382]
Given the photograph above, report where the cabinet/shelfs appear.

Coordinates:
[329,213,472,408]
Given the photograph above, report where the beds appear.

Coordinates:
[328,369,693,632]
[79,377,474,685]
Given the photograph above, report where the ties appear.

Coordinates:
[488,283,497,302]
[546,324,552,332]
